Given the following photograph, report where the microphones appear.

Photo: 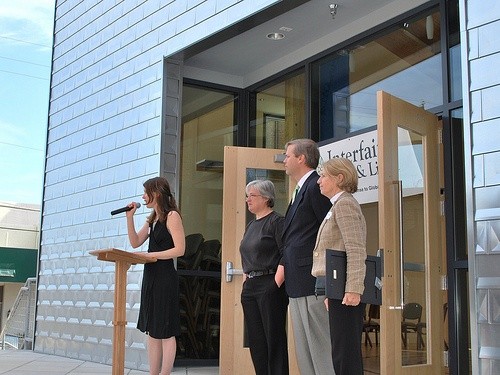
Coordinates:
[111,202,140,215]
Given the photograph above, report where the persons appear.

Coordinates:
[126,177,185,375]
[281,139,333,375]
[311,158,367,375]
[239,179,289,375]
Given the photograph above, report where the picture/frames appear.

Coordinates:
[263,113,286,148]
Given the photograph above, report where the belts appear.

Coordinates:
[246,269,275,278]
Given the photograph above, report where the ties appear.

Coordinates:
[291,185,300,205]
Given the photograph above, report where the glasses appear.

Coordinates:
[245,193,261,198]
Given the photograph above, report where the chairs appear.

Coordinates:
[362,301,451,356]
[175,233,223,360]
